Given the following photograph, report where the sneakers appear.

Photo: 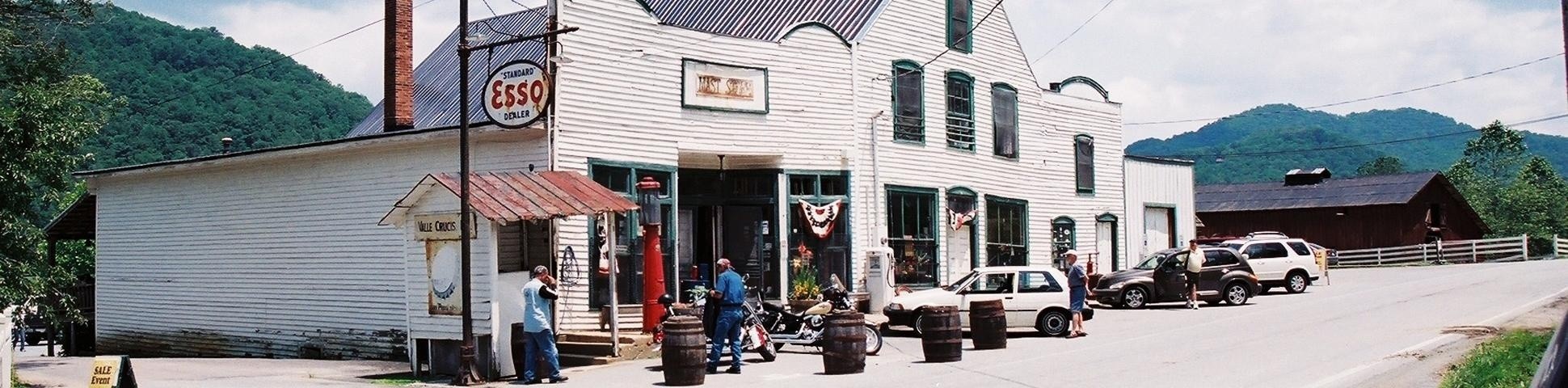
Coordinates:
[705,366,716,374]
[1065,334,1078,338]
[1194,302,1198,309]
[549,374,568,384]
[1075,331,1086,336]
[726,367,740,373]
[1185,302,1192,309]
[525,378,542,384]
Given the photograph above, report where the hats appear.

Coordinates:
[1063,249,1077,257]
[1189,239,1198,243]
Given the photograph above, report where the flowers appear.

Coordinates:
[788,243,824,301]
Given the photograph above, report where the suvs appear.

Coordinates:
[1218,237,1320,295]
[1244,231,1289,240]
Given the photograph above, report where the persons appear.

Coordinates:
[1180,237,1207,307]
[518,265,569,385]
[1065,250,1090,336]
[705,258,748,369]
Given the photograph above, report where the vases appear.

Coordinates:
[789,298,817,315]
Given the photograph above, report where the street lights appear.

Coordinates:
[635,175,668,332]
[448,0,580,386]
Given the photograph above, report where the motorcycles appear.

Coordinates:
[659,273,776,365]
[758,283,883,356]
[741,285,773,352]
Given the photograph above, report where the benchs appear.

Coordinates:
[600,303,646,331]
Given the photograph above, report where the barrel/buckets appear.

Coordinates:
[823,312,866,374]
[969,300,1007,350]
[662,316,706,385]
[920,305,962,362]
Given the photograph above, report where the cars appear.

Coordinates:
[1193,234,1244,245]
[1311,243,1328,272]
[1094,244,1263,307]
[1308,243,1340,266]
[884,265,1094,336]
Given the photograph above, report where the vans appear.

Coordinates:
[17,297,47,346]
